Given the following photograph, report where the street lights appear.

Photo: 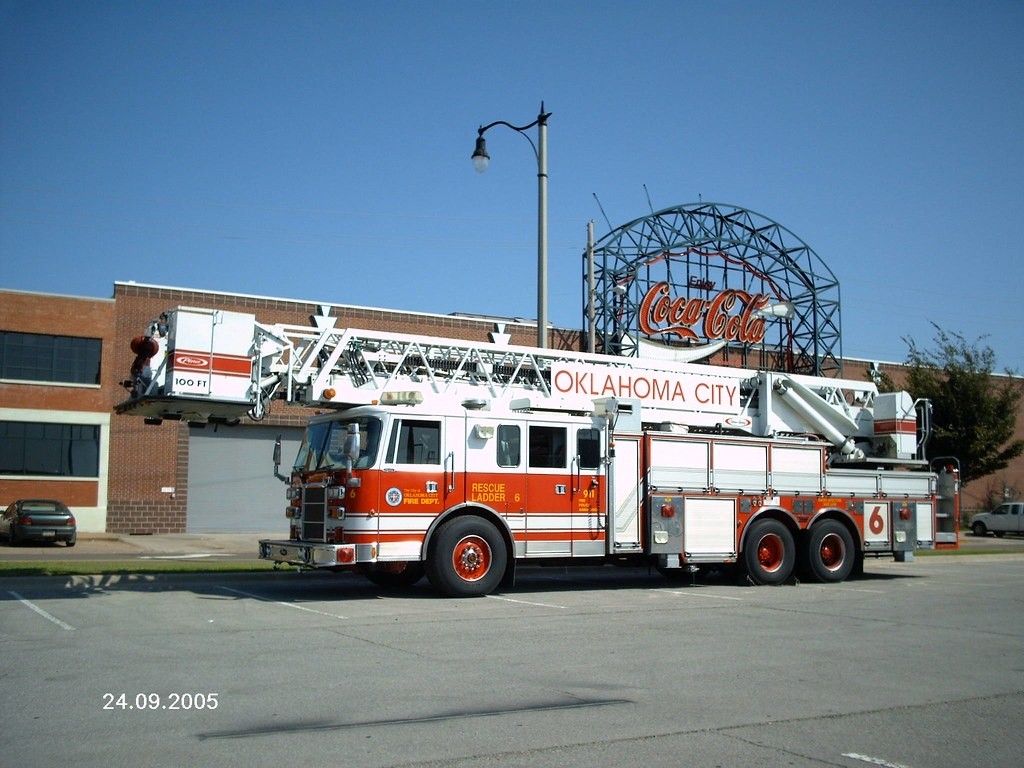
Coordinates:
[470,100,554,350]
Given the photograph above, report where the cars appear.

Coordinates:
[0,499,76,547]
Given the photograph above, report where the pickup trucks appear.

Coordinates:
[967,503,1024,538]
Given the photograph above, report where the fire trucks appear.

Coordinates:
[113,306,960,598]
